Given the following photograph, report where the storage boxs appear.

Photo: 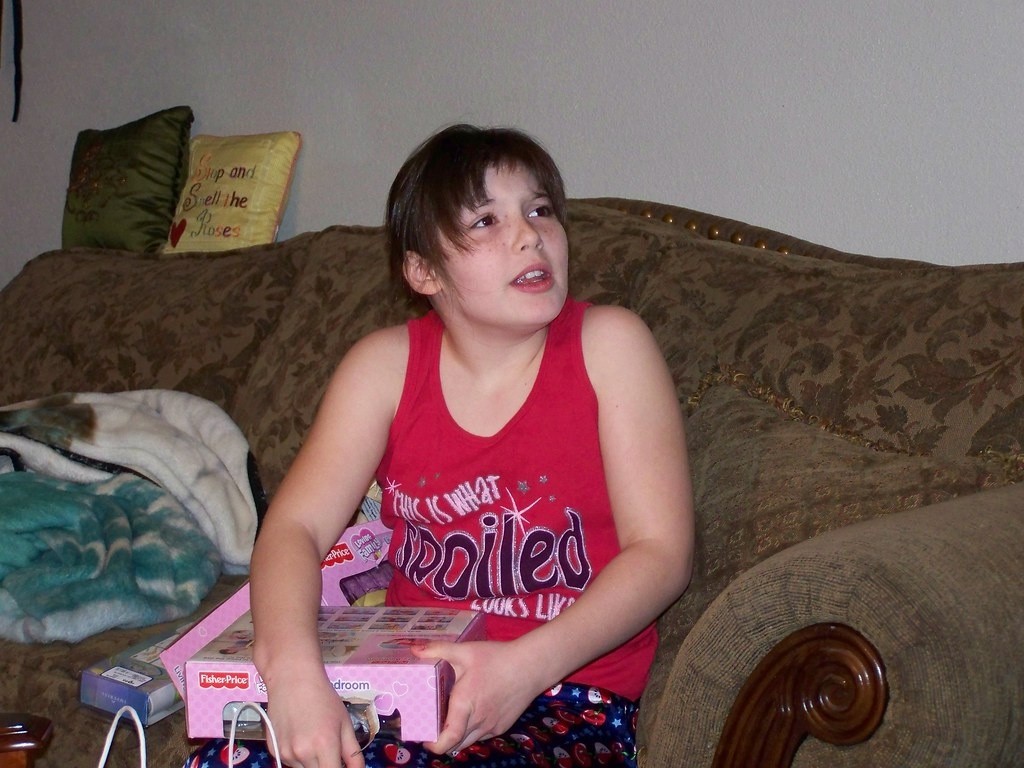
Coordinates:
[160,518,392,702]
[185,606,486,742]
[80,619,202,729]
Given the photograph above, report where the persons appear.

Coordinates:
[181,124,696,768]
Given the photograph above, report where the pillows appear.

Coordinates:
[163,134,300,254]
[62,106,194,252]
[635,364,1024,761]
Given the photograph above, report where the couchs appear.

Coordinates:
[0,198,1024,768]
